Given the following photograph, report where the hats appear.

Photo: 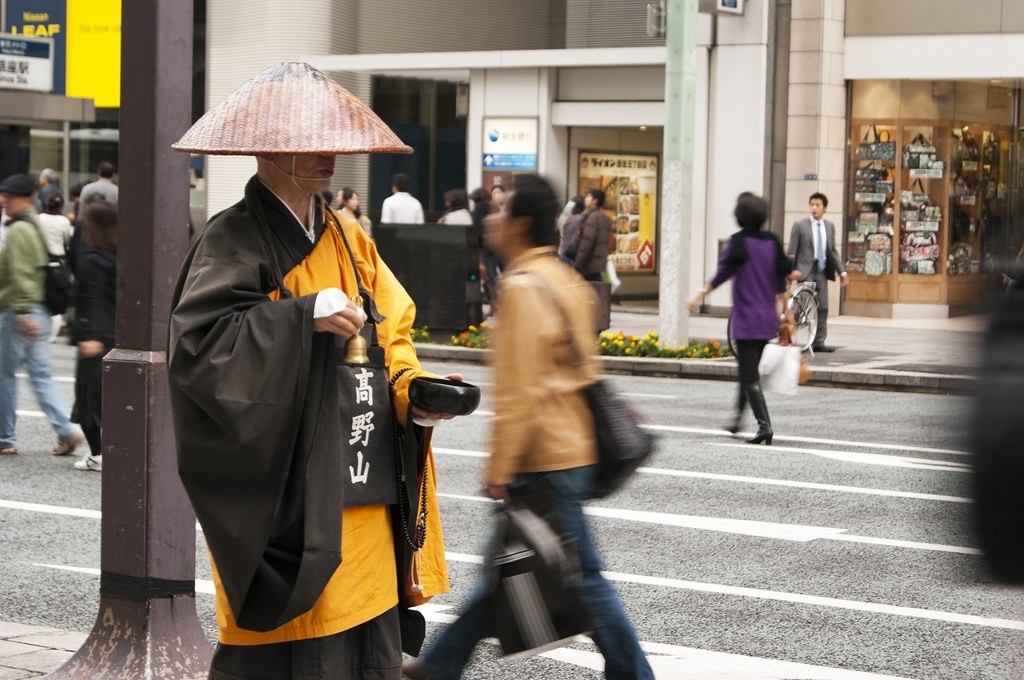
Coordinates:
[171,61,414,156]
[0,173,39,197]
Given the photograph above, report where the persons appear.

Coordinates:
[0,162,118,473]
[683,192,801,446]
[379,171,425,224]
[321,187,374,238]
[403,175,657,680]
[786,193,850,354]
[556,188,619,282]
[438,184,506,320]
[166,60,466,680]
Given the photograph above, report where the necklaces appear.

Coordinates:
[386,366,428,551]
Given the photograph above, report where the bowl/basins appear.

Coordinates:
[407,377,480,416]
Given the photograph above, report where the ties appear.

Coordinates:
[817,222,826,271]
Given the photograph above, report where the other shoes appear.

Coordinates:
[0,445,17,455]
[74,452,102,471]
[811,345,836,353]
[51,423,85,455]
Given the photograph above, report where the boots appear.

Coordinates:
[720,384,747,435]
[744,381,773,446]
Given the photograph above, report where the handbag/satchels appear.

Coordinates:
[757,328,810,397]
[43,258,72,318]
[583,380,654,499]
[483,500,593,660]
[601,260,620,294]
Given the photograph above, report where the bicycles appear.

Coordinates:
[726,268,820,364]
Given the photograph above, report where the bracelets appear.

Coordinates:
[702,289,708,294]
